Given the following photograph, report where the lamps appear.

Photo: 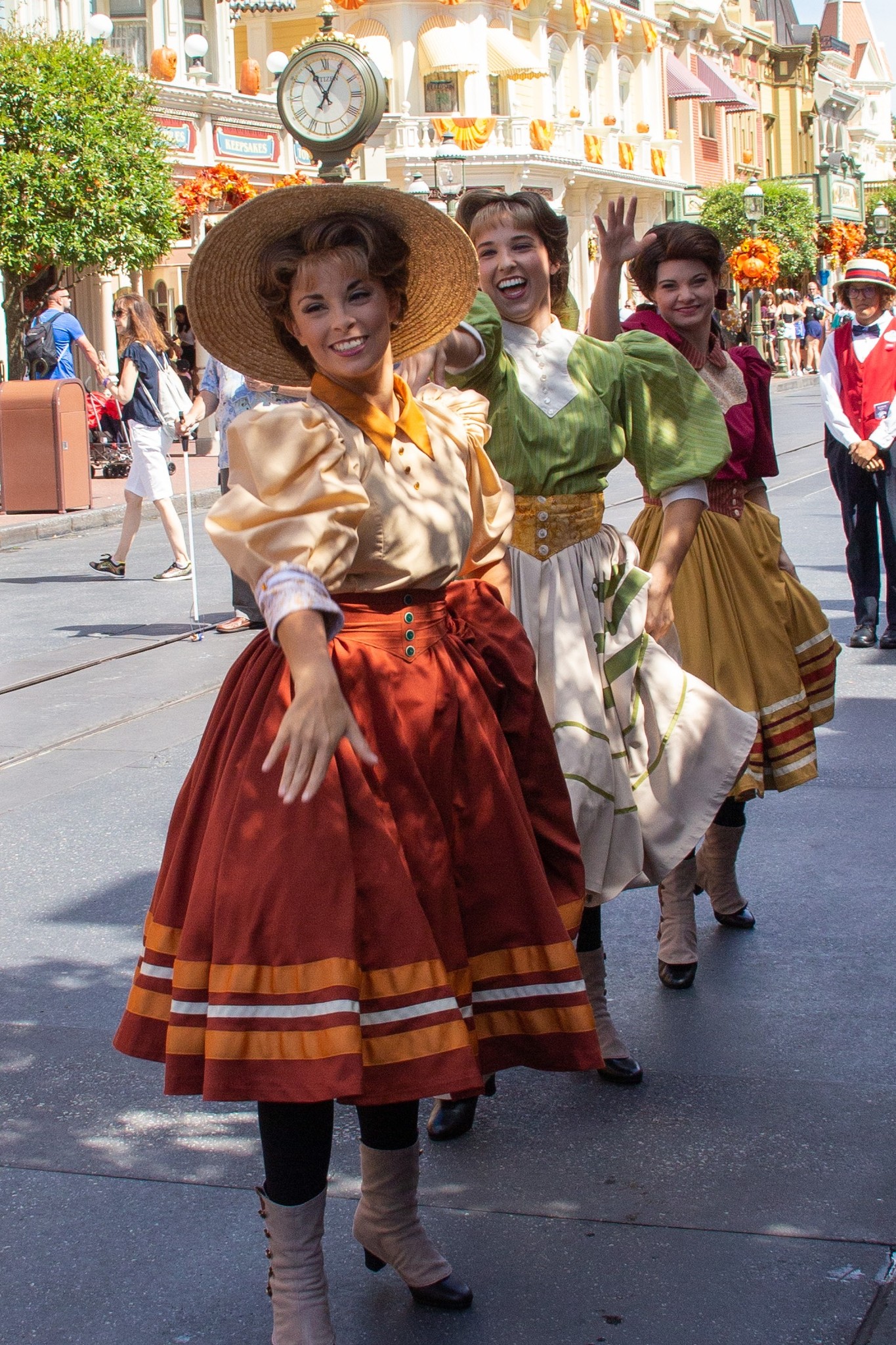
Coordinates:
[87,13,114,48]
[266,49,288,87]
[184,34,209,72]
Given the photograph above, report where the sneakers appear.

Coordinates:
[151,561,193,582]
[88,553,128,578]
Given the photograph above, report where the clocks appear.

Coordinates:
[274,42,387,150]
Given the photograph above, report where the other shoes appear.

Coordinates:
[770,361,779,368]
[792,370,803,376]
[802,366,820,374]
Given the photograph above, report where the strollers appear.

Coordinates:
[84,373,176,478]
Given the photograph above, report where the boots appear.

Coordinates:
[695,811,757,929]
[352,1131,473,1309]
[428,1074,498,1140]
[578,941,642,1086]
[656,857,700,990]
[256,1179,339,1345]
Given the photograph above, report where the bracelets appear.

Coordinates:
[103,378,111,388]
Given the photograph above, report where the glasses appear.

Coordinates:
[112,310,130,318]
[843,287,879,300]
[59,296,71,299]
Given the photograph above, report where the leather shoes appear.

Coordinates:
[880,624,896,649]
[215,617,267,633]
[850,624,877,647]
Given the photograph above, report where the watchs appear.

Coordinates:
[270,384,279,393]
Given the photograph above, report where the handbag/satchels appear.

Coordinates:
[735,332,747,343]
[777,318,786,328]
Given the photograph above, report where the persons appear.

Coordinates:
[114,180,604,1345]
[31,284,108,478]
[425,190,761,1144]
[740,282,844,379]
[816,255,896,650]
[172,353,308,635]
[588,195,842,988]
[150,304,198,444]
[89,294,195,582]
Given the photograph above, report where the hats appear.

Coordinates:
[177,357,189,373]
[182,184,480,390]
[832,259,896,298]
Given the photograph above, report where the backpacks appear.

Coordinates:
[814,306,824,319]
[25,313,70,373]
[122,341,199,442]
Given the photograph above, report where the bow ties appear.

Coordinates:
[852,324,881,338]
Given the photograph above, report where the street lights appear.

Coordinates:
[735,176,773,361]
[866,198,896,250]
